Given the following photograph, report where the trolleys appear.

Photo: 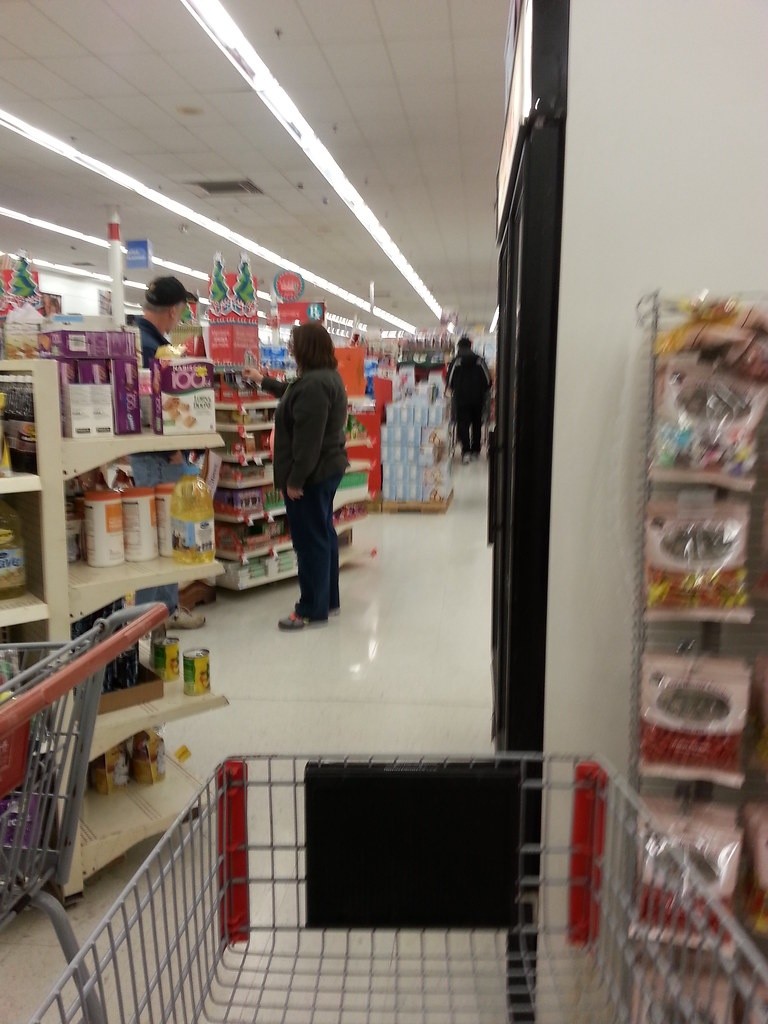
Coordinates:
[1,601,171,1024]
[452,390,492,461]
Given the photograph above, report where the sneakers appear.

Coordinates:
[141,632,151,646]
[168,603,205,628]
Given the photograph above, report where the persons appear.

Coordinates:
[123,276,209,632]
[240,321,352,634]
[448,336,495,463]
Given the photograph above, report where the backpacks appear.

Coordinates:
[456,355,481,406]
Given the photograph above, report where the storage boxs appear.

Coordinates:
[37,330,137,358]
[62,384,113,438]
[108,358,141,435]
[380,383,455,502]
[151,358,217,435]
[73,661,164,715]
[43,357,76,438]
[3,323,40,360]
[76,358,108,385]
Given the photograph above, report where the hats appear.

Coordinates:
[145,276,198,307]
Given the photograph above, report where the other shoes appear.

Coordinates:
[295,603,341,617]
[471,453,477,461]
[278,612,329,632]
[462,453,471,464]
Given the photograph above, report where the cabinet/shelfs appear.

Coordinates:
[0,357,231,908]
[213,396,377,590]
